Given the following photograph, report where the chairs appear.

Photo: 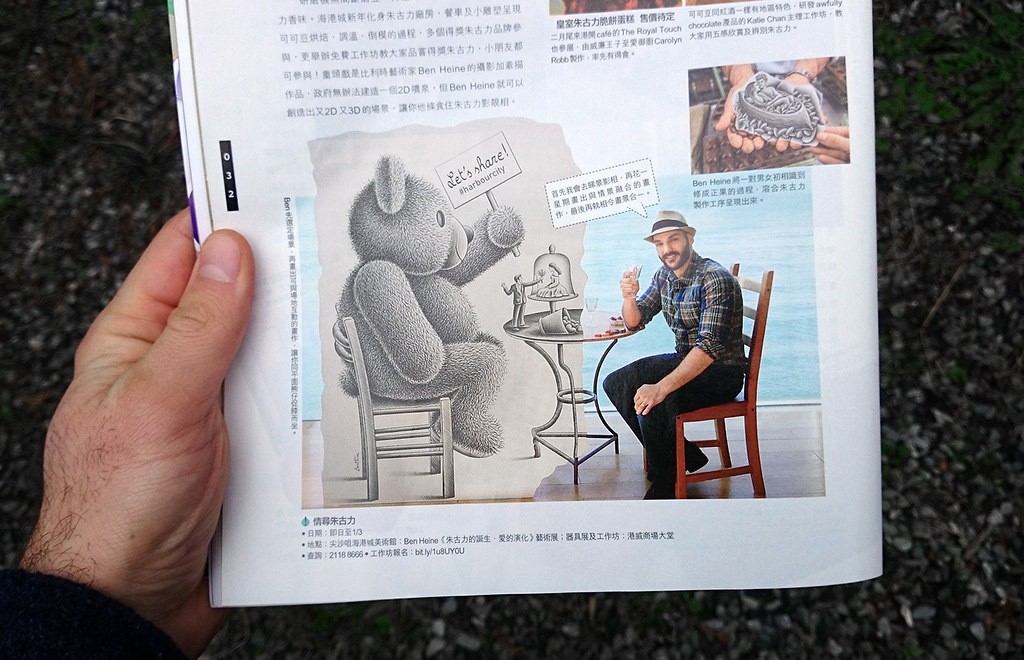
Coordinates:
[642,261,775,499]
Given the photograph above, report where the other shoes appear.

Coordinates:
[647,445,709,481]
[643,476,675,499]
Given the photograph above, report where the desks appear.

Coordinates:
[503,310,640,486]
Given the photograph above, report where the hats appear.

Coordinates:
[644,211,696,243]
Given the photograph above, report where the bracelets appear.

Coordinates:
[787,68,814,82]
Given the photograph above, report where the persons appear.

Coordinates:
[0,207,256,660]
[602,211,746,500]
[713,58,831,154]
[812,126,850,164]
